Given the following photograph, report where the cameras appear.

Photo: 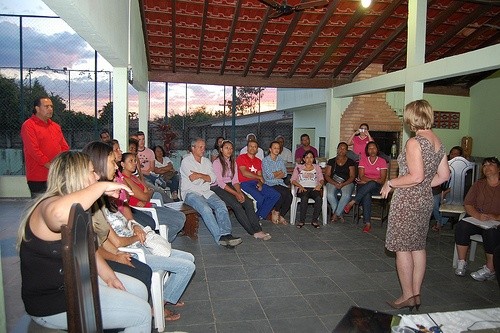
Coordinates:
[359,129,364,133]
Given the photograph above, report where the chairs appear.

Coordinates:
[60,156,500,333]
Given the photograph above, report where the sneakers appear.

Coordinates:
[470,264,495,281]
[344,206,349,213]
[455,259,468,275]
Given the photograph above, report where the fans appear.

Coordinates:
[258,0,328,18]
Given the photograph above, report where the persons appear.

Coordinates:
[236,141,281,225]
[20,96,70,199]
[82,130,182,318]
[325,142,356,225]
[177,137,243,249]
[213,139,273,241]
[344,141,387,232]
[295,134,318,165]
[17,150,152,333]
[83,141,196,321]
[211,133,292,163]
[346,123,374,160]
[260,142,294,226]
[289,150,325,229]
[432,146,463,231]
[380,99,451,311]
[455,156,500,282]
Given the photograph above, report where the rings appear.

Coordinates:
[381,187,383,190]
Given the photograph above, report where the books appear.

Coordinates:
[462,216,500,229]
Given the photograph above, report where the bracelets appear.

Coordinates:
[317,184,322,189]
[388,180,394,189]
[132,223,139,228]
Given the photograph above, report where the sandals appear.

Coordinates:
[296,222,304,228]
[311,222,320,229]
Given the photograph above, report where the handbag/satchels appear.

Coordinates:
[332,175,345,183]
[128,220,172,257]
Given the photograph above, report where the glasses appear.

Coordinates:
[222,140,233,146]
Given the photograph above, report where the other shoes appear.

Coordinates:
[271,210,279,224]
[279,216,288,225]
[362,225,370,232]
[347,209,350,210]
[218,235,242,246]
[164,309,180,320]
[222,241,243,249]
[258,215,264,226]
[338,216,345,223]
[165,299,184,307]
[331,214,338,223]
[431,223,441,232]
[171,193,177,199]
[253,232,271,240]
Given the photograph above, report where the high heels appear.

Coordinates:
[414,294,421,311]
[387,296,416,312]
